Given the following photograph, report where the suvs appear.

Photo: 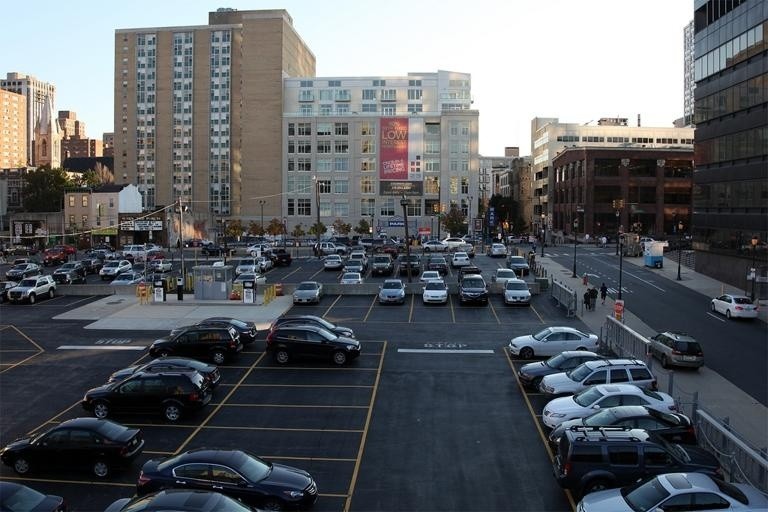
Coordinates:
[538,358,657,403]
[551,424,725,501]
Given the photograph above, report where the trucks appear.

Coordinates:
[640,237,670,251]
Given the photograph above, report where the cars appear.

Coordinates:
[509,325,600,360]
[576,471,768,512]
[517,350,608,391]
[542,383,677,431]
[547,404,696,453]
[680,233,692,241]
[711,294,759,320]
[590,231,611,243]
[647,330,705,371]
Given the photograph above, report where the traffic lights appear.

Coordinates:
[619,199,625,209]
[613,200,618,209]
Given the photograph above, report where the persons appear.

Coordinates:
[600,283,607,304]
[584,288,591,312]
[533,240,536,253]
[590,286,598,311]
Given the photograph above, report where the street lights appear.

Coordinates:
[671,212,676,234]
[370,213,374,258]
[618,235,625,300]
[615,209,619,256]
[431,212,434,240]
[752,235,758,302]
[311,175,325,261]
[466,195,473,235]
[259,200,266,243]
[541,212,545,258]
[572,219,579,278]
[221,217,228,264]
[400,191,414,284]
[481,211,486,254]
[677,221,684,281]
[175,203,191,291]
